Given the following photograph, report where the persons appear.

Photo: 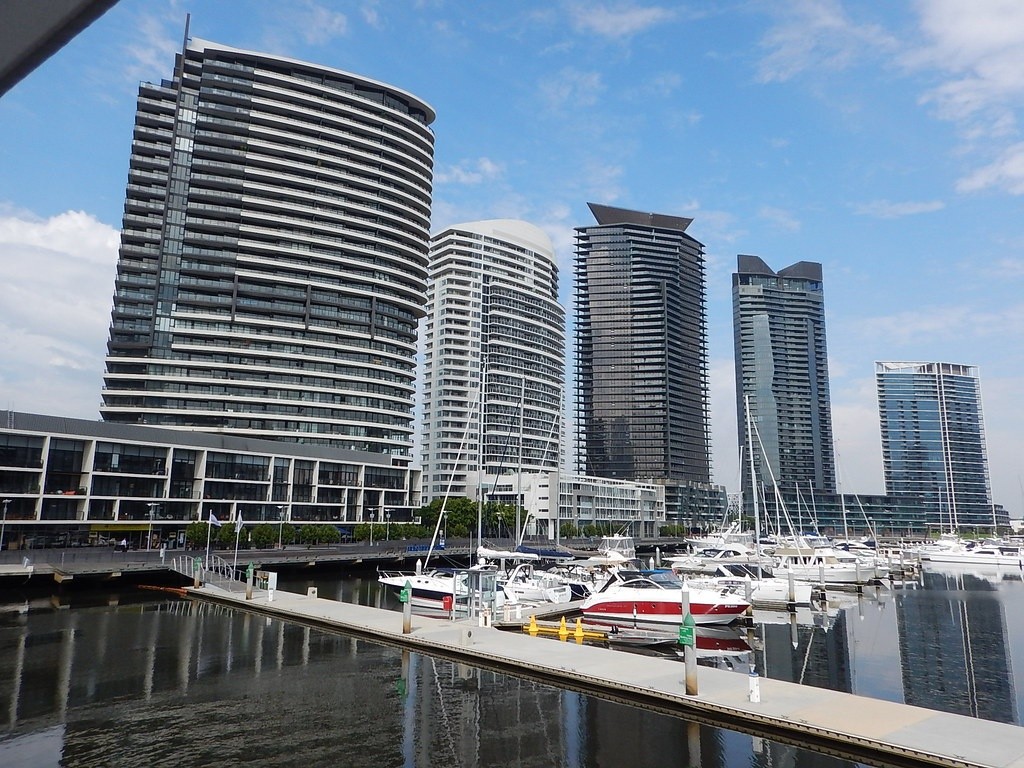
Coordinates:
[159,546,163,564]
[186,541,194,550]
[437,535,445,550]
[120,537,126,552]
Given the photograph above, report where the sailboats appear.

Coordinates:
[377,357,1024,650]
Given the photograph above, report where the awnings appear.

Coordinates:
[338,528,349,534]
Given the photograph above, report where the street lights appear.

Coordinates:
[277,506,289,549]
[147,503,160,549]
[367,508,377,546]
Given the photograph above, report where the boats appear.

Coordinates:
[580,616,753,658]
